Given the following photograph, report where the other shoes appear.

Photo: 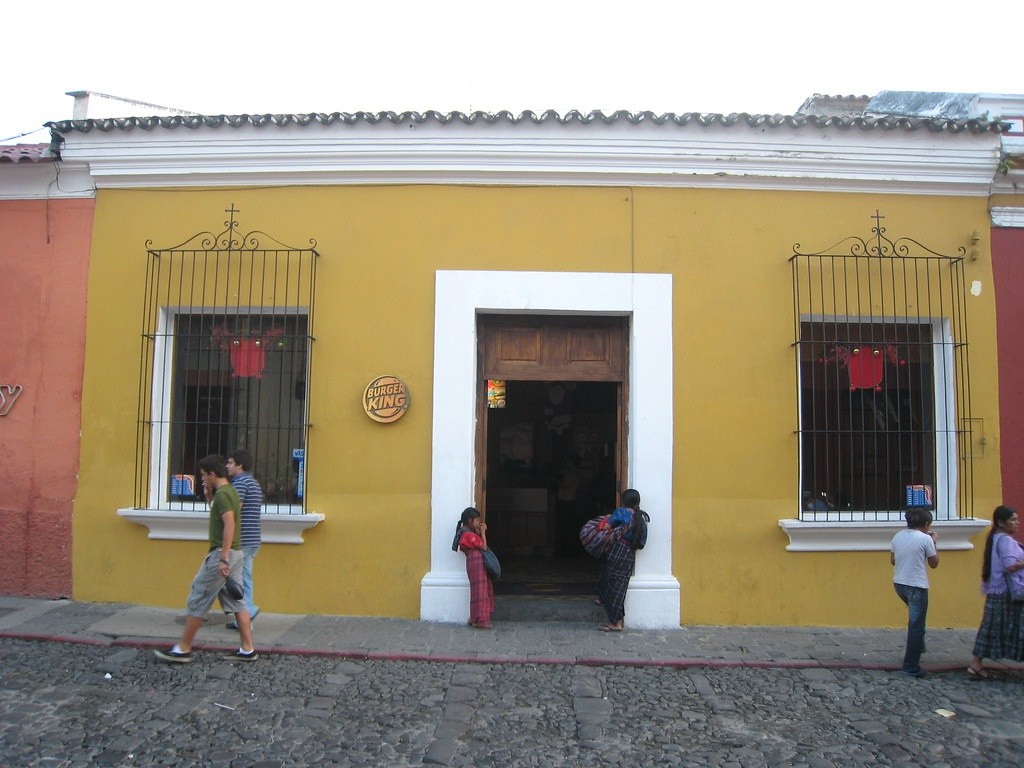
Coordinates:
[153,644,195,663]
[603,624,623,632]
[222,646,258,662]
[226,608,260,630]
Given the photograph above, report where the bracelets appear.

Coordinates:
[934,542,936,544]
[220,559,229,565]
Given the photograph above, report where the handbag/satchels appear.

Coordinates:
[996,537,1024,601]
[482,547,501,579]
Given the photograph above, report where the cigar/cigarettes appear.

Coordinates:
[202,483,205,485]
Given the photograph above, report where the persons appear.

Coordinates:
[452,507,494,629]
[593,489,644,632]
[889,507,939,677]
[154,450,263,663]
[967,505,1024,678]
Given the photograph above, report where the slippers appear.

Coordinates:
[901,666,927,678]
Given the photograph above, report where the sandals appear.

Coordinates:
[967,666,992,680]
